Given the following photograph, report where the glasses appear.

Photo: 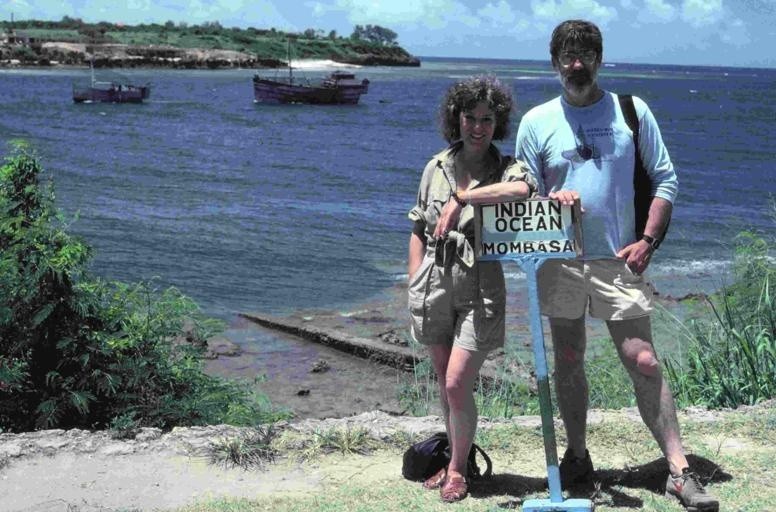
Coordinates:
[555,51,599,67]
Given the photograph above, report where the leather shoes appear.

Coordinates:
[423,467,476,487]
[442,475,470,501]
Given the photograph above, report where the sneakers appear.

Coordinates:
[560,448,594,488]
[665,473,719,512]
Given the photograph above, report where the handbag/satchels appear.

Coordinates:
[618,94,669,248]
[402,432,492,482]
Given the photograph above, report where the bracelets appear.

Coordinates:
[451,190,469,208]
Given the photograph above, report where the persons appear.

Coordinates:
[514,18,723,512]
[405,72,543,505]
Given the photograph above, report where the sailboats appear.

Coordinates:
[72,58,149,104]
[250,39,368,105]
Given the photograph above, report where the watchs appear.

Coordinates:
[642,233,662,251]
